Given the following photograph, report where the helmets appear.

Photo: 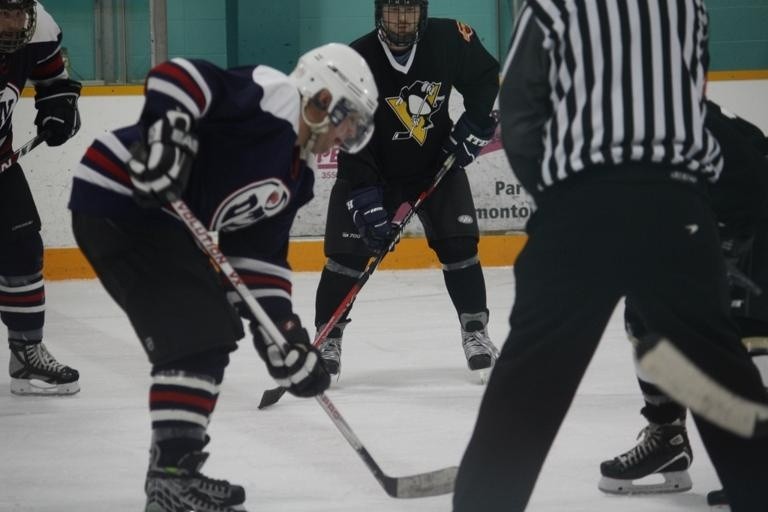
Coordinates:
[374,0,429,46]
[288,42,379,154]
[0,0,37,56]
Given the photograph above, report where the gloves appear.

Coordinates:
[439,112,497,169]
[249,314,330,396]
[124,109,200,208]
[34,79,82,146]
[344,185,400,257]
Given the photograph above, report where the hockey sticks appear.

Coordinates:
[132,144,459,501]
[260,108,501,411]
[129,142,459,499]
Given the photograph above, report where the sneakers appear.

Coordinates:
[315,318,352,375]
[460,308,501,370]
[144,434,245,512]
[600,421,692,480]
[9,337,79,384]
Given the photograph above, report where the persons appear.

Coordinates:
[449,1,768,512]
[597,96,768,483]
[310,1,502,378]
[1,1,84,385]
[67,40,380,512]
